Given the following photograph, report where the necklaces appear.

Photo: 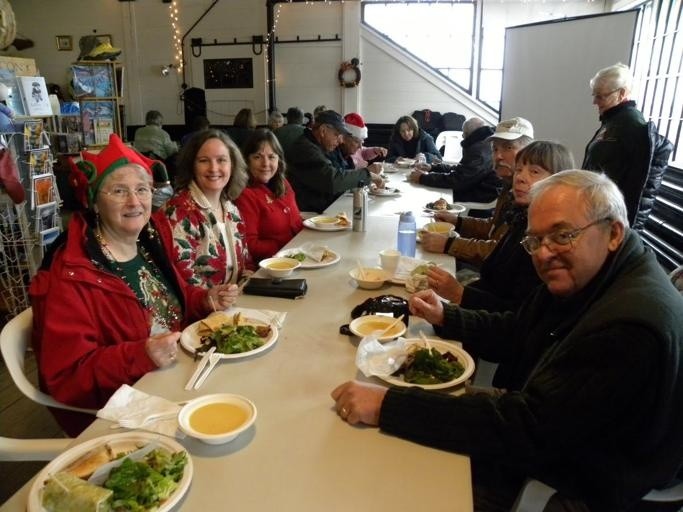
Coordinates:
[206,200,222,212]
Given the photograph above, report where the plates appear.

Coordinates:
[423,202,465,213]
[364,186,400,196]
[302,214,351,232]
[276,248,340,270]
[370,335,475,390]
[416,229,460,243]
[180,313,277,358]
[383,168,398,173]
[23,432,194,511]
[396,162,415,166]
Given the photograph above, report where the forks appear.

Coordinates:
[194,353,224,389]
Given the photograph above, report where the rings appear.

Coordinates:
[166,350,176,360]
[340,403,349,416]
[434,279,439,288]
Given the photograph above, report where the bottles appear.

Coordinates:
[352,180,368,232]
[394,211,416,257]
[419,154,426,163]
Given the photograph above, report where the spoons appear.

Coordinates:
[373,313,404,337]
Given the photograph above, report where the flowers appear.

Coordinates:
[337,58,362,89]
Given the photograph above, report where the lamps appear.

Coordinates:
[157,60,186,81]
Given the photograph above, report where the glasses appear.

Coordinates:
[94,186,157,203]
[519,218,613,256]
[591,88,622,101]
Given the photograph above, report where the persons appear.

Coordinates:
[145,128,257,293]
[407,118,504,202]
[417,115,535,264]
[134,111,210,159]
[422,139,579,314]
[238,128,303,271]
[585,63,647,226]
[328,166,683,509]
[26,134,240,440]
[385,116,442,164]
[234,105,387,214]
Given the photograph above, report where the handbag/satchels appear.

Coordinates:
[242,277,306,299]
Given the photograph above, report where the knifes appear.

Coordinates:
[184,346,215,390]
[109,411,180,429]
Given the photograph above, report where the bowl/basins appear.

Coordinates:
[349,314,406,340]
[349,267,392,289]
[310,217,340,227]
[177,392,256,445]
[259,258,300,277]
[424,221,454,236]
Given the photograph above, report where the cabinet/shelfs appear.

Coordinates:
[49,48,125,171]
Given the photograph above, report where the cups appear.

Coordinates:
[377,249,400,275]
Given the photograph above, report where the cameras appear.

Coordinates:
[365,296,410,327]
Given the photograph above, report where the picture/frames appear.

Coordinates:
[54,35,72,52]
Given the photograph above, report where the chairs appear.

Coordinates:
[432,128,464,166]
[0,161,681,512]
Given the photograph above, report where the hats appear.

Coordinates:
[483,117,534,142]
[315,110,367,138]
[66,133,170,212]
